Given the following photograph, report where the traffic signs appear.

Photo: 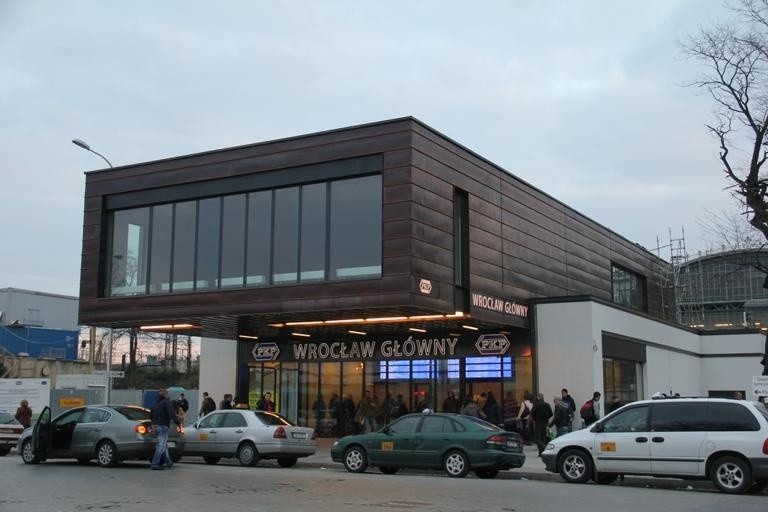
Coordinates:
[108,371,124,378]
[752,375,768,397]
[87,380,108,387]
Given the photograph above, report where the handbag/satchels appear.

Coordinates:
[521,407,529,418]
[359,416,365,424]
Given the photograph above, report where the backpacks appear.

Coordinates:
[581,400,594,417]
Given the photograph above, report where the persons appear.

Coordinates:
[357,390,433,432]
[734,391,743,400]
[15,399,32,429]
[150,386,276,470]
[312,394,326,437]
[328,393,355,438]
[443,391,501,427]
[502,388,626,457]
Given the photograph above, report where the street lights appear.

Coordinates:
[80,339,95,375]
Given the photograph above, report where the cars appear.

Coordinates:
[170,409,316,468]
[0,407,25,457]
[293,409,342,435]
[331,407,525,478]
[17,404,184,468]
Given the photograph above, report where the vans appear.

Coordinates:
[541,397,767,495]
